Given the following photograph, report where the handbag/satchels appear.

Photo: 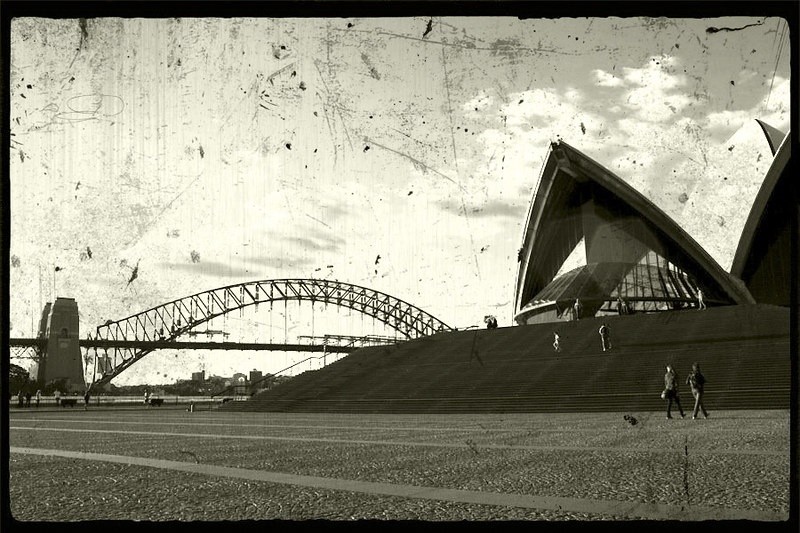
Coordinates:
[660,389,668,398]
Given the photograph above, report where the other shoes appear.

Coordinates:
[693,417,696,420]
[666,417,673,420]
[704,413,710,419]
[681,412,686,419]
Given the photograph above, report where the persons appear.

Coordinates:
[553,331,561,354]
[598,322,612,352]
[696,286,707,311]
[484,315,498,329]
[615,297,633,315]
[686,363,710,420]
[665,365,687,420]
[143,389,157,406]
[16,387,89,413]
[575,299,581,322]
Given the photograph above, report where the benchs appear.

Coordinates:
[148,398,164,405]
[60,400,76,408]
